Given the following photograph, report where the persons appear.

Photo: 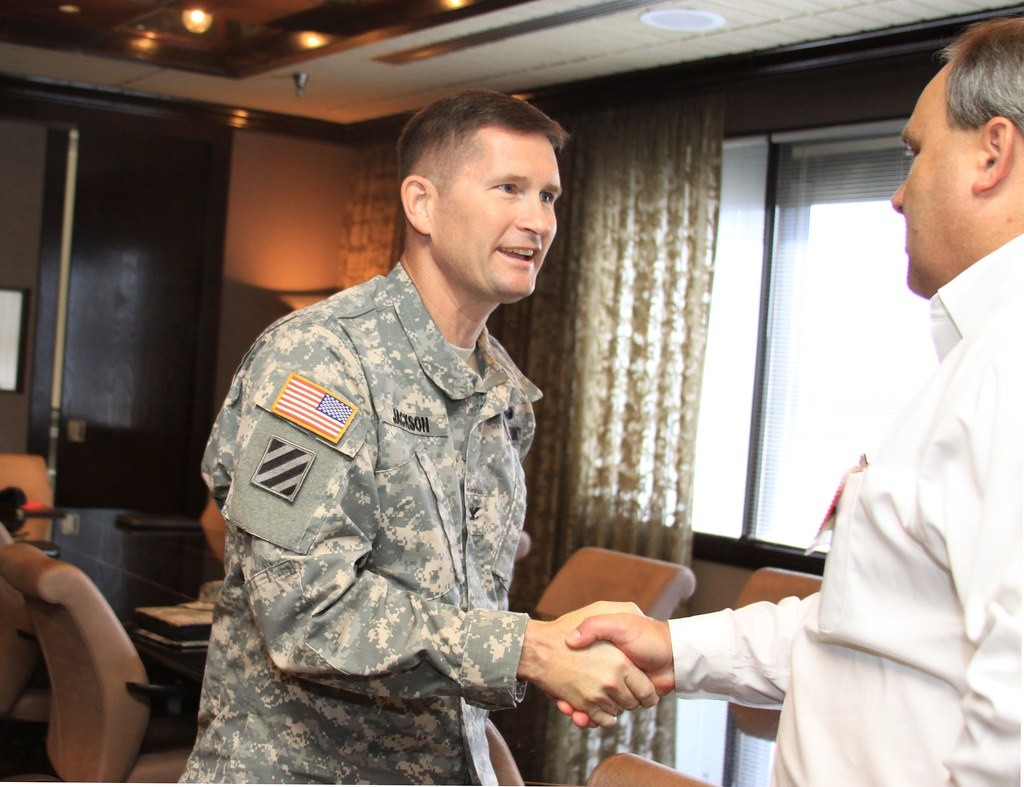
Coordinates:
[559,13,1021,787]
[172,91,659,785]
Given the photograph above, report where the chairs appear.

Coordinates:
[0,452,827,782]
